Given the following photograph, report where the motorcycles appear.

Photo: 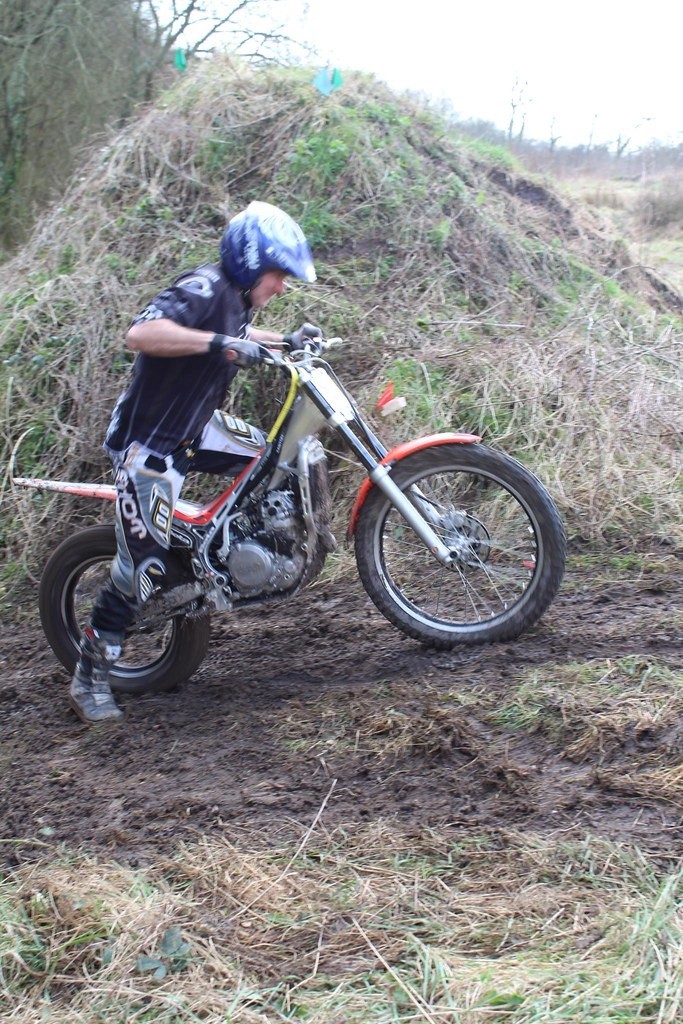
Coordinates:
[8,334,566,702]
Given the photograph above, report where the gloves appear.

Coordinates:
[282,322,322,353]
[209,333,260,370]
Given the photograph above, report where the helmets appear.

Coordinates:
[220,200,317,290]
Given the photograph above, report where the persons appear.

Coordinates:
[60,197,325,725]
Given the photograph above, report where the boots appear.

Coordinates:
[67,619,123,725]
